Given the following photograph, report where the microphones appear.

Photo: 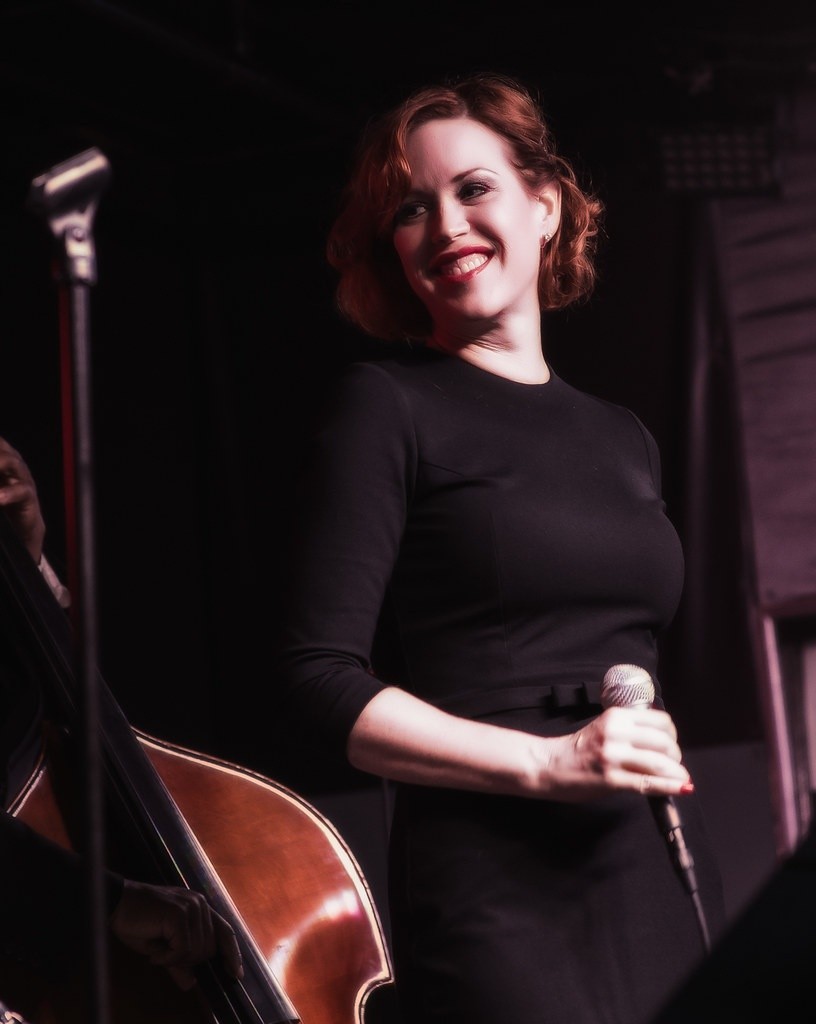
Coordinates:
[600,664,697,896]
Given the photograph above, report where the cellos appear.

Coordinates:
[1,504,399,1023]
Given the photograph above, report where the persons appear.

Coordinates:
[0,439,246,1024]
[279,73,721,1024]
